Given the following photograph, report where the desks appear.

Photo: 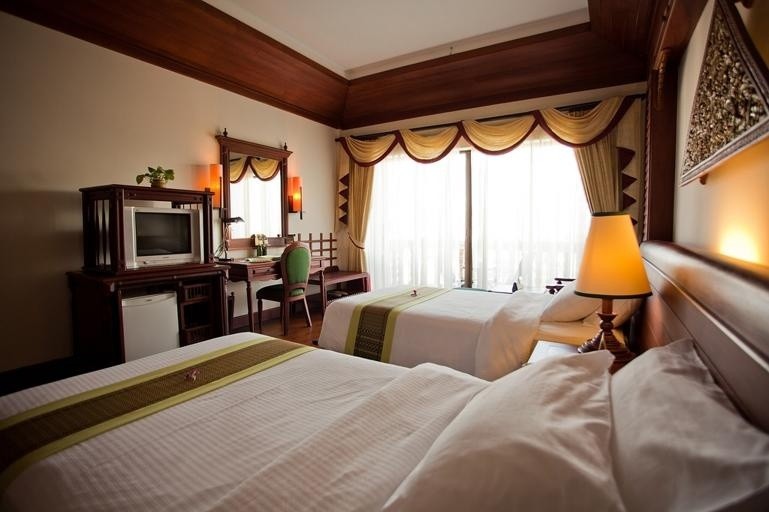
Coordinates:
[216,255,327,335]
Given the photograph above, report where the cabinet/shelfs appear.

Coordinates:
[72,269,227,361]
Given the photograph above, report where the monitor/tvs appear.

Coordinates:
[124,205,201,270]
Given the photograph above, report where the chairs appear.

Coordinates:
[257,242,313,336]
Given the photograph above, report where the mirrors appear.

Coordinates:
[214,127,292,249]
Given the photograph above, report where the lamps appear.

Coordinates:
[289,177,303,220]
[573,211,652,361]
[209,164,229,221]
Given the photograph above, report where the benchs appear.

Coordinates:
[294,233,372,321]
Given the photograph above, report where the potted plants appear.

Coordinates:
[137,165,176,187]
